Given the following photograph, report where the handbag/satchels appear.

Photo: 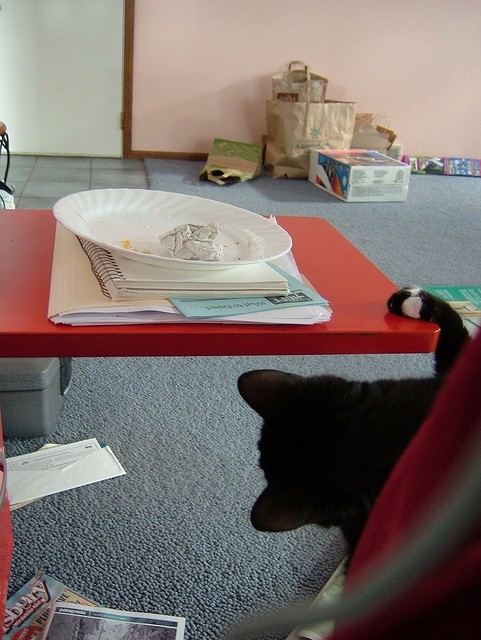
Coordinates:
[272,61,330,103]
[265,65,358,179]
[200,138,265,186]
[352,114,392,155]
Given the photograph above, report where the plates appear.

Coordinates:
[53,188,293,269]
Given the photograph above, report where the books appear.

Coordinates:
[47,217,333,326]
[413,284,480,319]
[2,572,187,640]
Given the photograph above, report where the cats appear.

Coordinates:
[235,284,471,577]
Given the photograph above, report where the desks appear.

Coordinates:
[0,208,440,357]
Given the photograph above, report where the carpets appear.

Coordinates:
[1,157,480,637]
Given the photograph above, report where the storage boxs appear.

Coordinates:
[2,359,73,438]
[308,149,412,202]
